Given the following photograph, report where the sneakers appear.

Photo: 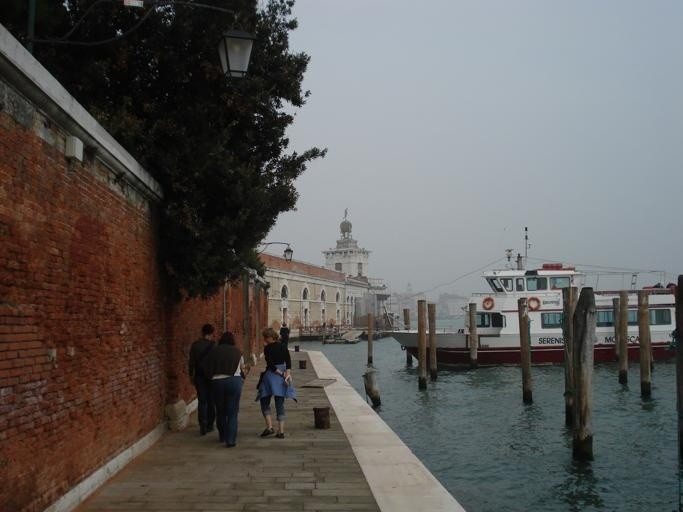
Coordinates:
[200,428,215,434]
[219,438,235,447]
[261,427,284,438]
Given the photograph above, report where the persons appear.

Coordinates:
[186,322,220,438]
[279,322,290,348]
[253,326,298,440]
[207,331,246,449]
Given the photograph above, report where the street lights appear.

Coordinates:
[256,243,293,264]
[26,0,255,82]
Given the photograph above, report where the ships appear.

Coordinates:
[382,227,678,369]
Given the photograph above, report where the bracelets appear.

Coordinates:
[280,371,284,377]
